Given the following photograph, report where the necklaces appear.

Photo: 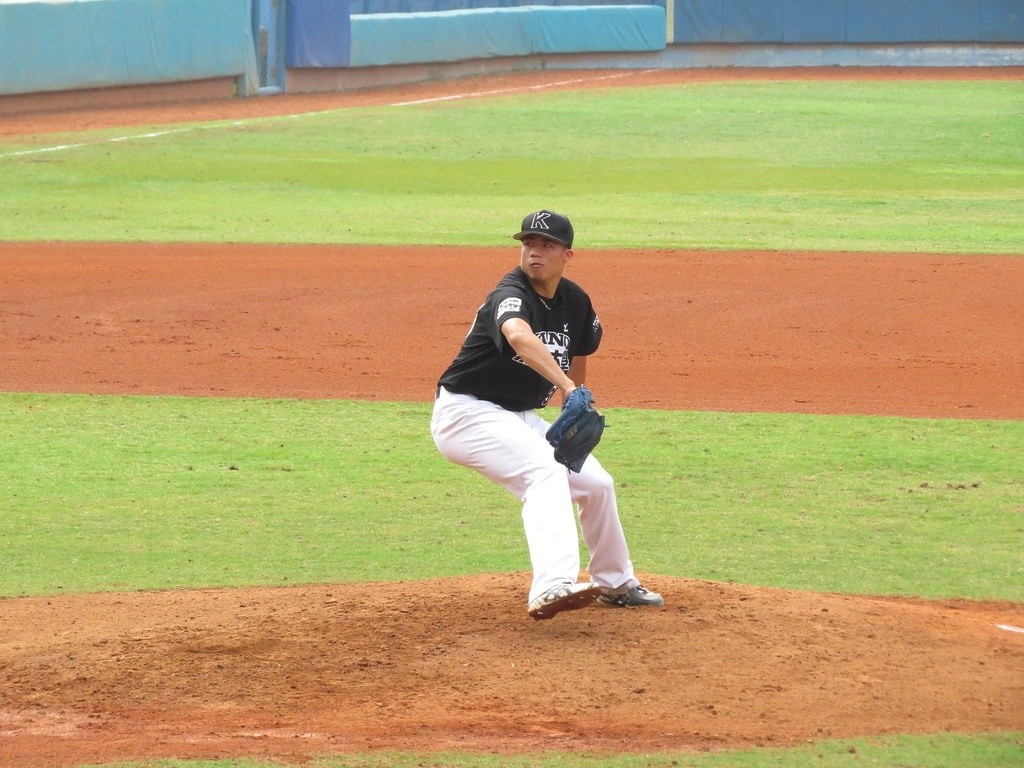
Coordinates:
[539,295,553,311]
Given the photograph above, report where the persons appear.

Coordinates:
[432,210,666,621]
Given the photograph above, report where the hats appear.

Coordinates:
[513,208,574,249]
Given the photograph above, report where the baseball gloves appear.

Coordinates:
[547,387,606,472]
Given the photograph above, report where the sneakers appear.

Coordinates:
[528,581,601,621]
[597,584,664,608]
